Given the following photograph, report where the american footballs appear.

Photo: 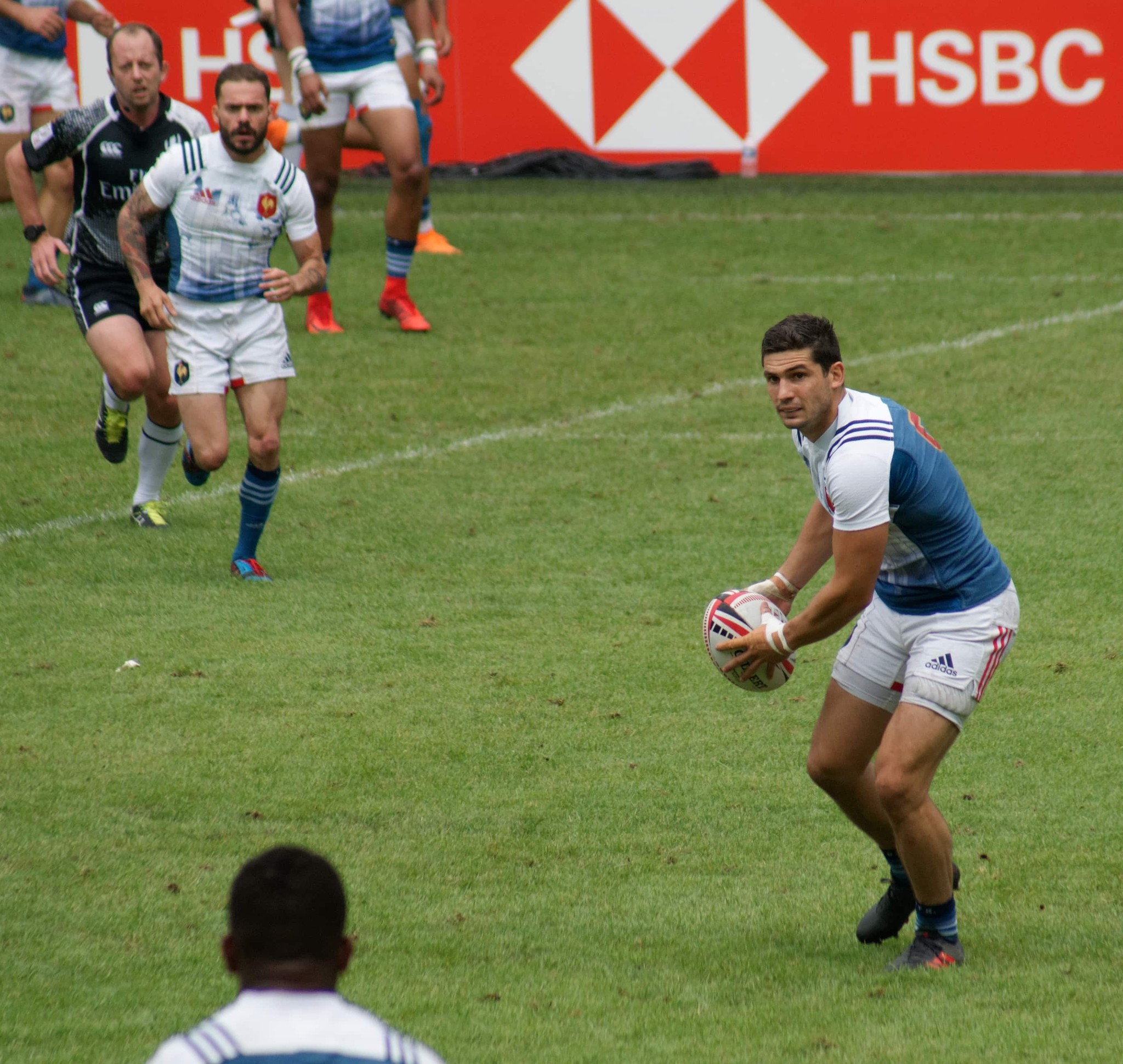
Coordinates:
[702,589,795,692]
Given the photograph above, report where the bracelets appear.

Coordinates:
[762,613,796,658]
[749,572,799,605]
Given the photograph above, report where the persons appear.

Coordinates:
[115,62,327,584]
[273,1,447,336]
[150,843,445,1061]
[244,0,458,254]
[2,21,211,526]
[713,314,1018,971]
[0,1,121,307]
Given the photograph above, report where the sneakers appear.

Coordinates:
[413,227,462,257]
[95,387,131,465]
[182,440,210,486]
[879,932,965,975]
[305,293,344,335]
[20,283,78,308]
[856,862,963,945]
[230,559,273,583]
[129,499,171,529]
[379,276,432,332]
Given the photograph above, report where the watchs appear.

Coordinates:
[23,224,45,241]
